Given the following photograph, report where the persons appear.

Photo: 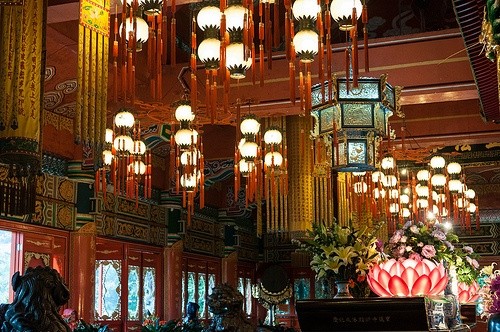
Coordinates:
[183,302,204,332]
[62,308,79,332]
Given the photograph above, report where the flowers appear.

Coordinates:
[200,321,206,328]
[291,212,391,283]
[174,320,184,332]
[474,262,500,319]
[141,310,172,332]
[388,219,480,273]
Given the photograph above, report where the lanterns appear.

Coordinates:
[112,0,176,102]
[1,102,480,234]
[189,0,371,123]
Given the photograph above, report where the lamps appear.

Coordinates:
[197,3,223,70]
[180,151,197,192]
[113,108,134,157]
[221,2,253,79]
[292,0,321,62]
[191,149,202,184]
[128,139,146,176]
[330,0,362,31]
[175,96,199,149]
[238,101,476,219]
[119,0,149,52]
[102,128,114,166]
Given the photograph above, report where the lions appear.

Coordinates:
[2,265,72,332]
[207,283,256,332]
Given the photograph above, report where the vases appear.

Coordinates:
[428,259,448,330]
[445,266,461,325]
[333,282,354,300]
[488,314,500,332]
